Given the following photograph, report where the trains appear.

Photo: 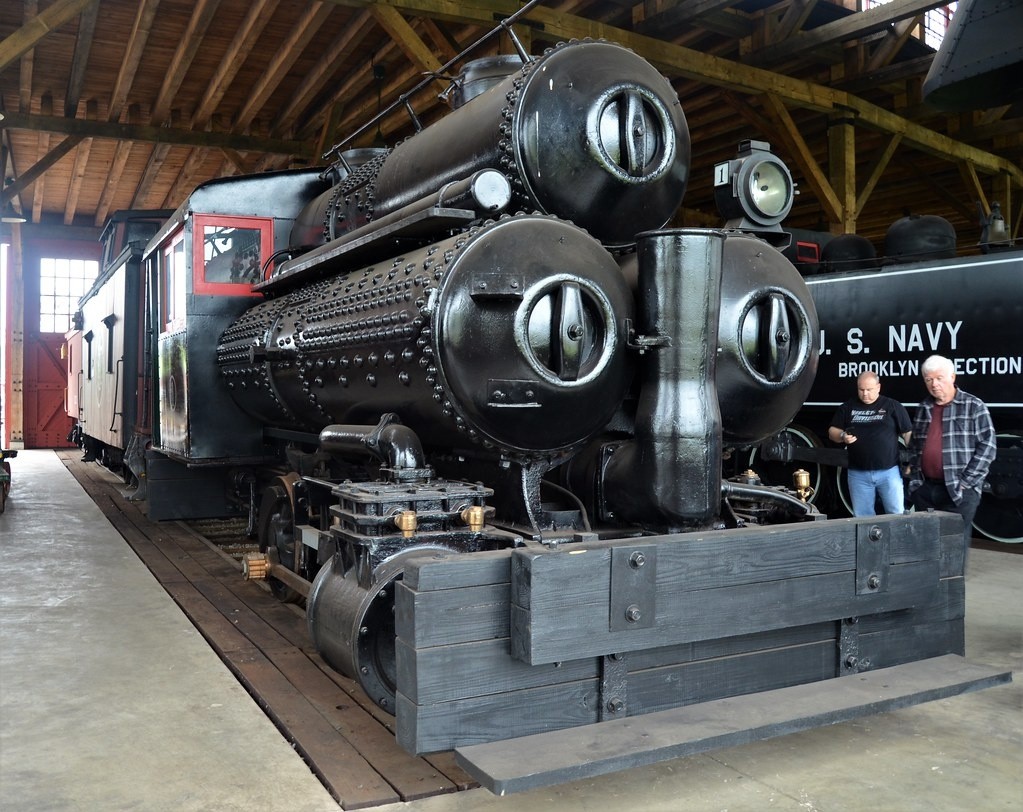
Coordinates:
[64,39,1016,797]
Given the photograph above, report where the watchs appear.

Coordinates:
[840,432,846,442]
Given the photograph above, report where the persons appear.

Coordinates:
[829,371,913,518]
[903,355,996,573]
